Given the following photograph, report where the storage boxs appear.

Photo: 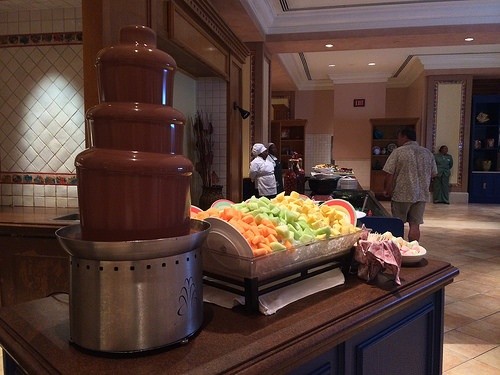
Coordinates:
[283,158,306,196]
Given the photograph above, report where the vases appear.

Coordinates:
[199,185,225,211]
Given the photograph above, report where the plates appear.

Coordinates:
[191,206,201,217]
[203,217,256,274]
[319,200,357,227]
[355,211,366,218]
[211,199,236,209]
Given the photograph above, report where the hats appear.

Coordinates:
[266,142,275,148]
[251,143,267,157]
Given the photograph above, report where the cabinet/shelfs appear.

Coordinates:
[370,117,420,198]
[270,119,305,177]
[0,236,459,374]
[468,94,500,203]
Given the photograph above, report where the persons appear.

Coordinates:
[431,145,453,204]
[249,142,283,199]
[382,127,438,242]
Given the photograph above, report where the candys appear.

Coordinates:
[191,191,359,256]
[367,231,422,256]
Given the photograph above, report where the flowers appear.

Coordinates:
[193,113,215,187]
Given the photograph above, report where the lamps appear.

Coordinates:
[234,102,250,119]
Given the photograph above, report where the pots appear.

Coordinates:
[307,175,341,195]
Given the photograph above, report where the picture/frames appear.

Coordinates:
[429,80,466,188]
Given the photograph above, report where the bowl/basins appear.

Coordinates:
[400,246,426,263]
